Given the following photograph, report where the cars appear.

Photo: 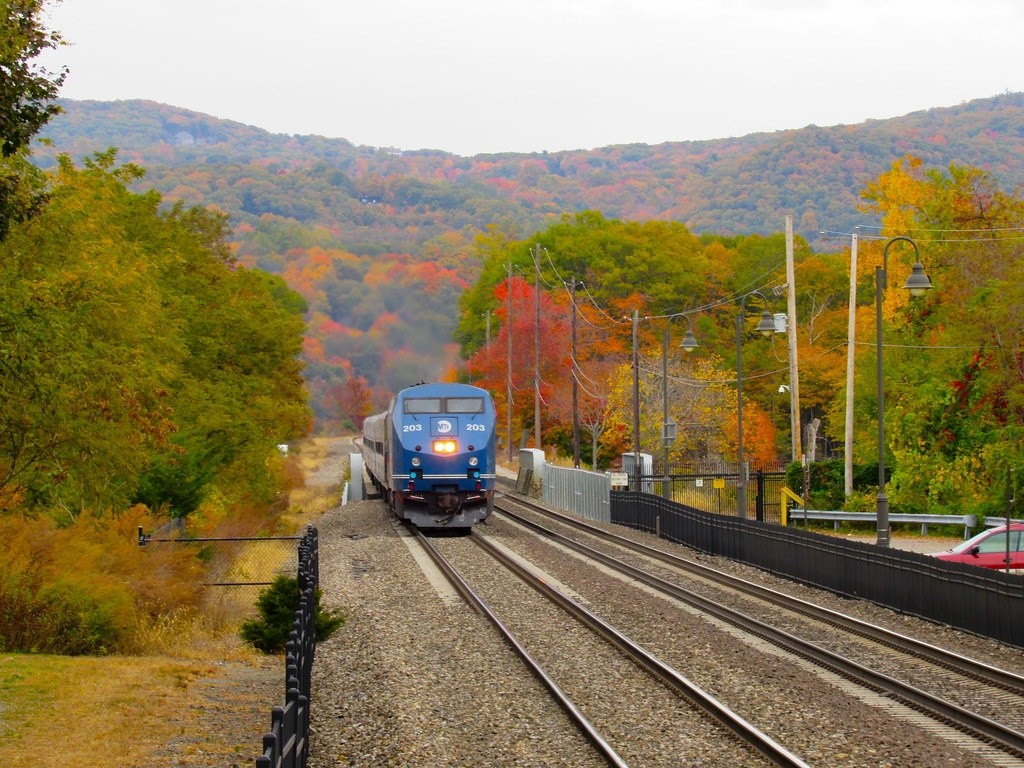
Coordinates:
[922,522,1024,576]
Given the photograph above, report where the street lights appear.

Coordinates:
[875,238,935,549]
[734,290,778,523]
[662,311,699,501]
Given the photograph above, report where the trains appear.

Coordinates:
[359,380,497,534]
